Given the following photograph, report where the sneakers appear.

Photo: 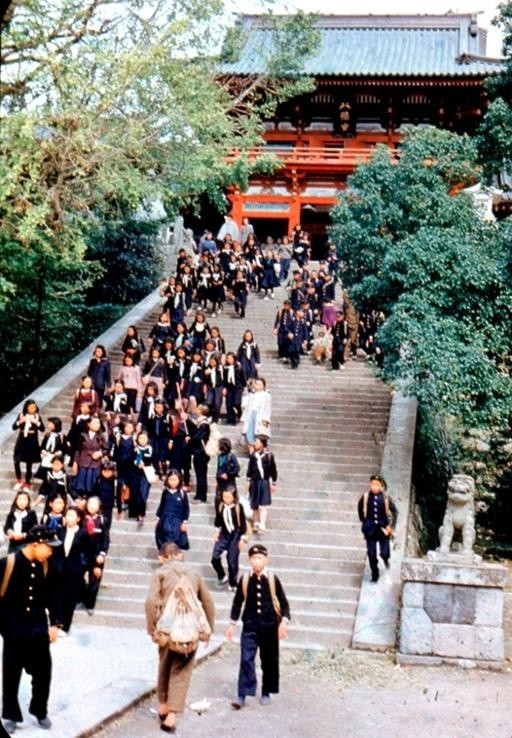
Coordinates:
[159,712,176,731]
[232,696,244,708]
[216,576,236,592]
[59,628,71,638]
[39,717,51,729]
[371,577,377,585]
[3,719,16,732]
[261,695,271,705]
[380,555,390,568]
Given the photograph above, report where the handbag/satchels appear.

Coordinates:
[143,464,156,484]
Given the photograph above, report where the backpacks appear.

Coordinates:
[197,420,224,457]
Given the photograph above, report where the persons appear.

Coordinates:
[2,492,111,633]
[226,544,291,708]
[357,474,398,581]
[11,313,279,587]
[145,541,215,733]
[0,526,63,734]
[157,216,390,372]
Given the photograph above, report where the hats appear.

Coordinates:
[25,525,62,547]
[248,545,268,557]
[370,475,383,482]
